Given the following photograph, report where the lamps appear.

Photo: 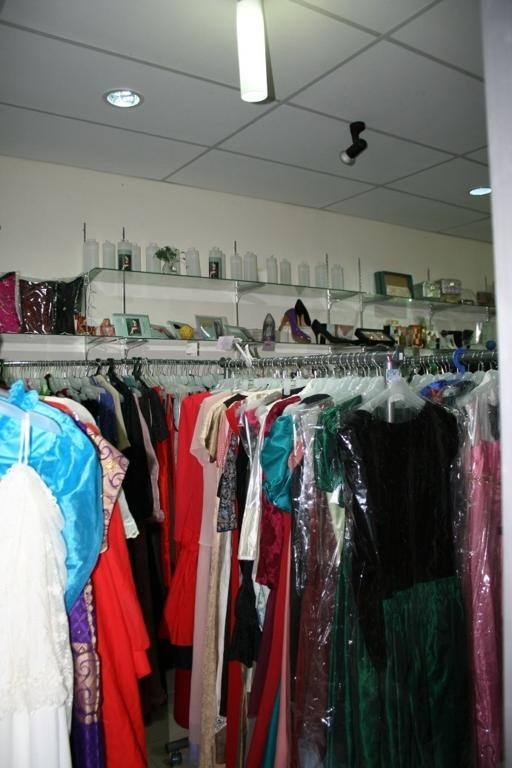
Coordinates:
[236,0,269,105]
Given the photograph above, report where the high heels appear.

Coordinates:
[295,299,311,326]
[262,314,276,342]
[312,319,351,345]
[278,309,312,343]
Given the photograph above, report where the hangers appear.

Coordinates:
[0,355,218,392]
[220,356,501,421]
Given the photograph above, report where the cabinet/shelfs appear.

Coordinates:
[73,268,496,348]
